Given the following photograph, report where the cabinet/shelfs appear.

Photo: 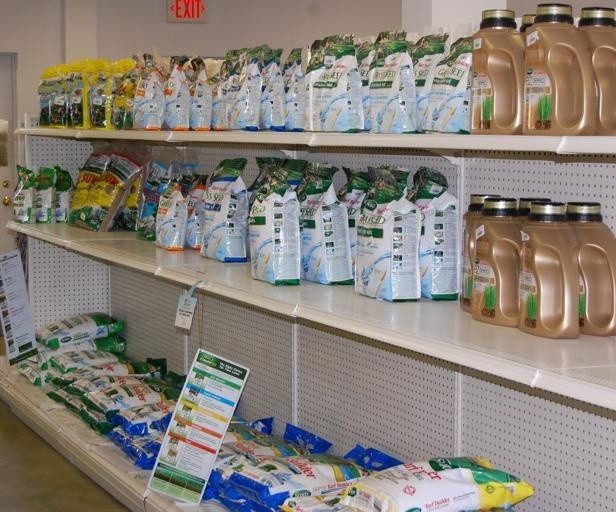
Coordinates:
[0,126,616,512]
